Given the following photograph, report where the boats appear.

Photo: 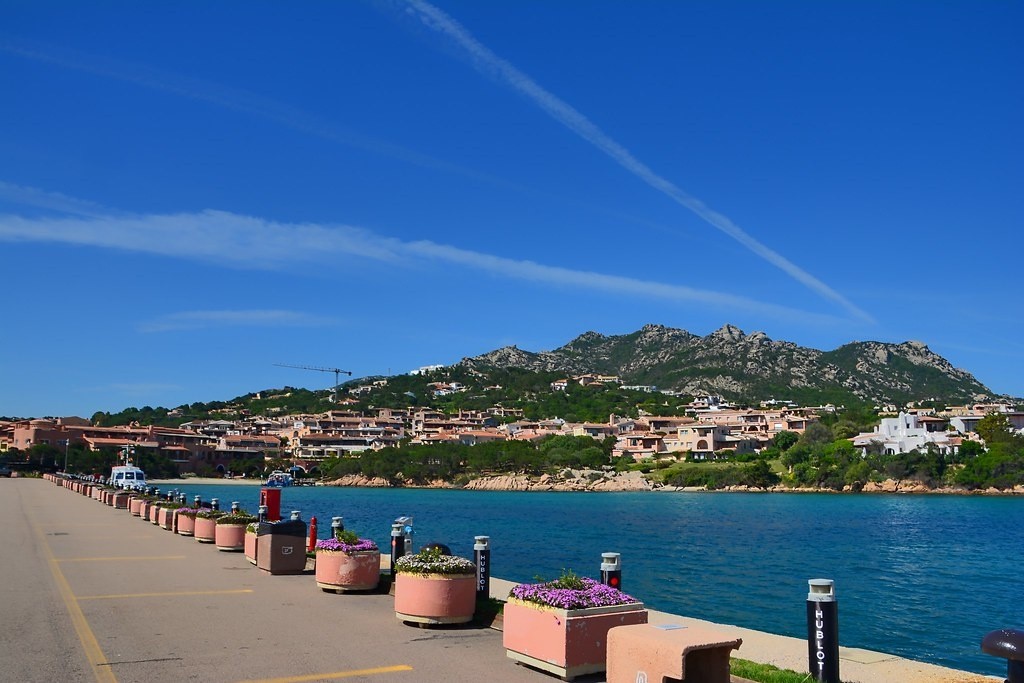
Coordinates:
[110,466,147,490]
[267,470,293,488]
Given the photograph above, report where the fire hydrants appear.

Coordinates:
[307,516,318,551]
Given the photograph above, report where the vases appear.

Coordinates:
[395,572,477,629]
[178,514,196,536]
[315,549,380,594]
[42,472,125,505]
[194,518,220,543]
[215,523,248,552]
[127,497,132,512]
[130,497,144,516]
[172,509,183,534]
[140,503,151,521]
[113,494,128,508]
[150,506,161,525]
[159,507,174,531]
[502,596,649,682]
[244,532,258,565]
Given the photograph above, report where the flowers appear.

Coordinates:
[177,505,210,521]
[215,507,257,525]
[128,493,140,498]
[142,497,159,506]
[172,503,200,510]
[394,546,477,577]
[505,566,640,612]
[136,493,151,500]
[115,488,135,495]
[196,508,228,520]
[314,528,379,556]
[149,500,173,508]
[245,519,282,536]
[160,501,183,511]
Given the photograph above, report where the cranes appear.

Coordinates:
[272,362,352,401]
